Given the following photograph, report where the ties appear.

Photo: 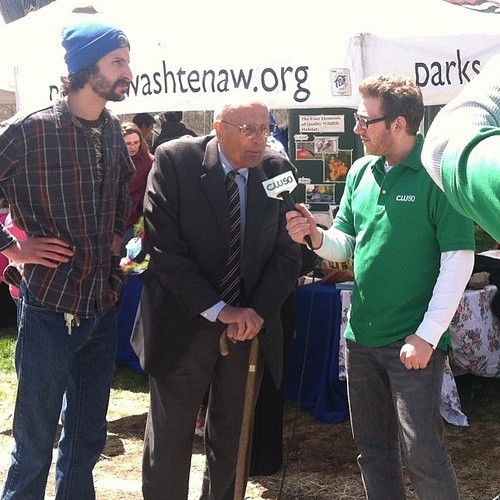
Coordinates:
[216,171,241,308]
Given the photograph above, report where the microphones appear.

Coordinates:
[260,157,316,252]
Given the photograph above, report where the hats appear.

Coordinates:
[61,24,130,75]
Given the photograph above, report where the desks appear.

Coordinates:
[115,248,500,426]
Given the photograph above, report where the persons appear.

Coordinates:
[286,71,476,500]
[153,111,197,155]
[133,113,157,139]
[121,122,154,228]
[421,50,500,243]
[0,22,136,500]
[128,93,303,500]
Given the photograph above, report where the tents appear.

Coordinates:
[0,0,500,116]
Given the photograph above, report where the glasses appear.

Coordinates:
[353,112,409,128]
[126,140,140,148]
[220,120,276,138]
